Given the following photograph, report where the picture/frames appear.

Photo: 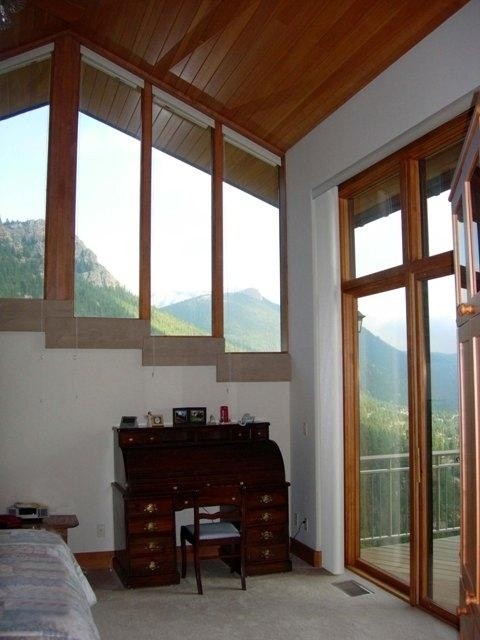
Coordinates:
[171,406,207,426]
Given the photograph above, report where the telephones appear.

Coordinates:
[241,413,254,423]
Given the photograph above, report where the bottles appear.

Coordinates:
[220,406,229,422]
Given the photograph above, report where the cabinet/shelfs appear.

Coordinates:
[109,424,296,590]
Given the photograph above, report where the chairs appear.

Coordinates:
[180,484,250,599]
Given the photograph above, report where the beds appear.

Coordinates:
[0,512,102,639]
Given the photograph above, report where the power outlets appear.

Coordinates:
[295,514,310,534]
